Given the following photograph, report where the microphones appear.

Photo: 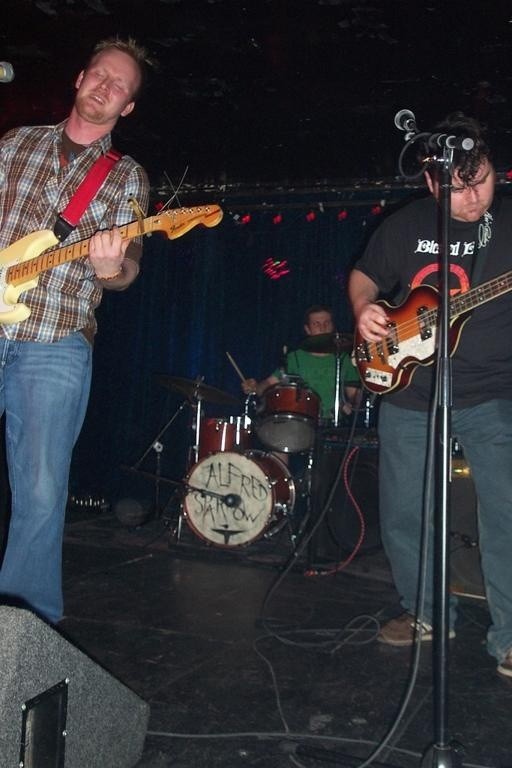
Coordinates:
[392,104,417,133]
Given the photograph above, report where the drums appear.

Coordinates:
[182,448,296,549]
[188,416,252,470]
[254,381,321,454]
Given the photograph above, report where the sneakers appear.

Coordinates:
[497,652,512,677]
[377,612,456,647]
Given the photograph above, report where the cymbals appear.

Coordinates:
[298,334,354,352]
[166,377,240,404]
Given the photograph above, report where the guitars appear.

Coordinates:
[351,270,512,395]
[1,204,223,325]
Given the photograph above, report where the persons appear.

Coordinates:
[0,33,157,622]
[237,304,364,420]
[348,110,512,678]
